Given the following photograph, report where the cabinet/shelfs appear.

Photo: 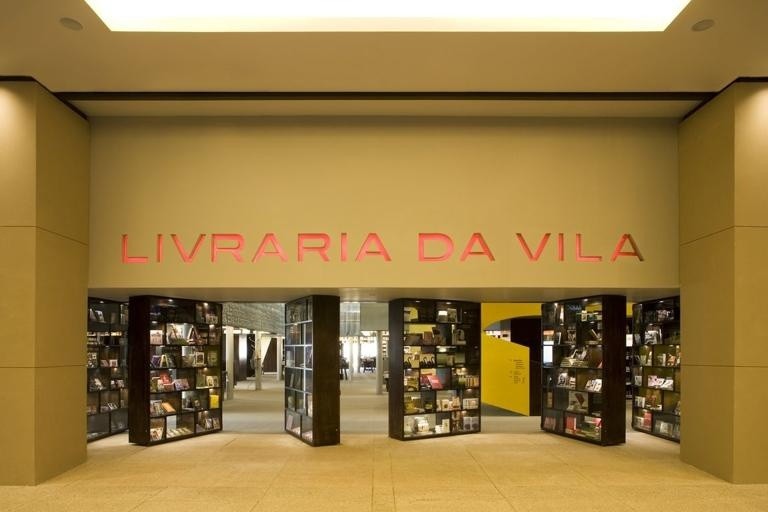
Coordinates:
[630,295,684,443]
[284,295,341,448]
[539,293,627,449]
[86,296,130,443]
[131,295,223,445]
[385,296,484,442]
